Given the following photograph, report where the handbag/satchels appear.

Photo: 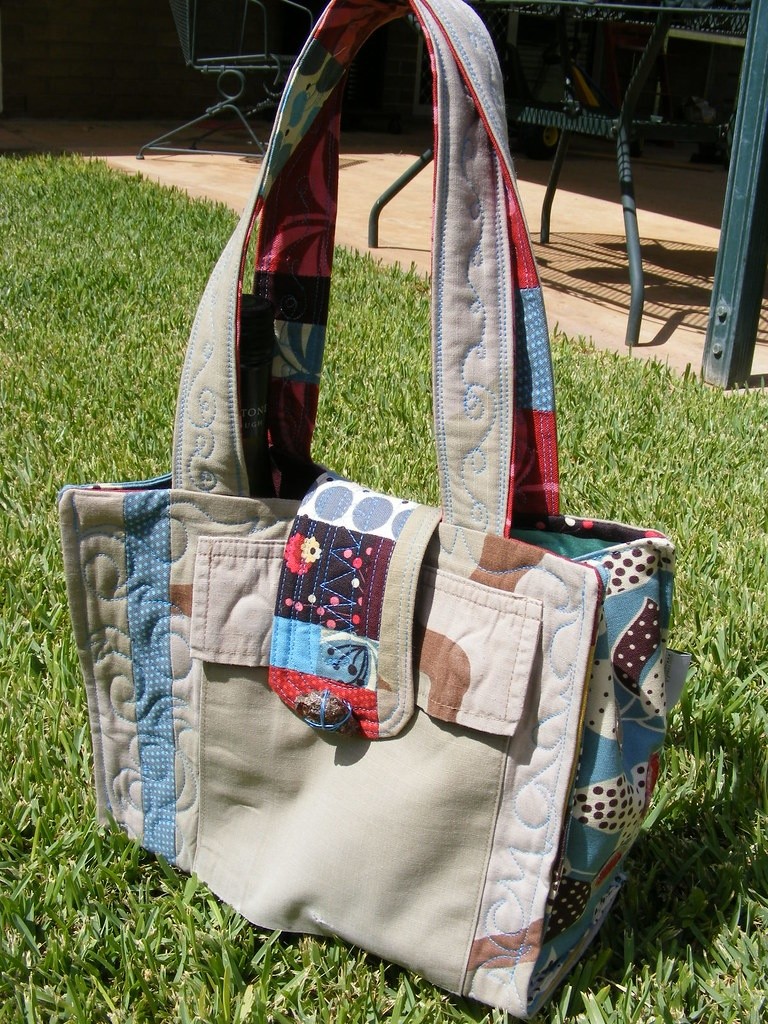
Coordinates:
[59,0,692,1022]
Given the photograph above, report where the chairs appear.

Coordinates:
[369,0,754,347]
[137,0,358,166]
